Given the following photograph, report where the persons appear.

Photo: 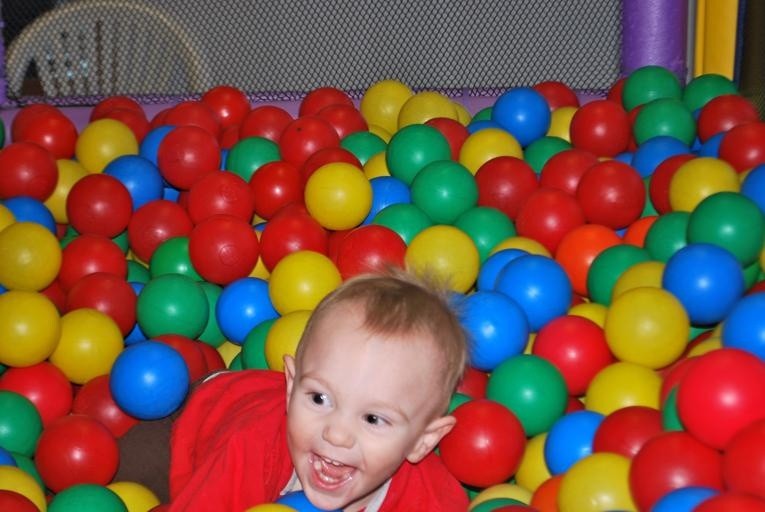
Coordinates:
[104,253,483,510]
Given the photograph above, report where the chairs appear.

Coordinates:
[8,3,211,104]
